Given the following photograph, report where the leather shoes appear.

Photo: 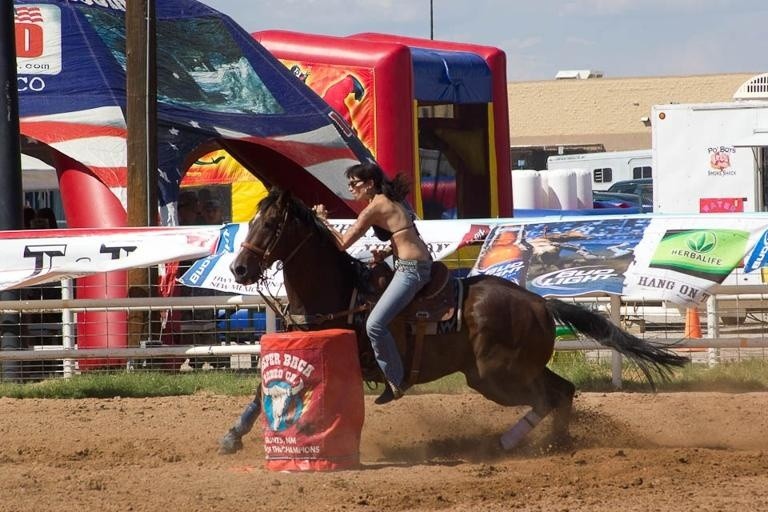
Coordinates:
[374,379,413,404]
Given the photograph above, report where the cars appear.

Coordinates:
[591,190,653,208]
[608,178,653,204]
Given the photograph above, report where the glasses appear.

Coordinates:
[348,180,362,187]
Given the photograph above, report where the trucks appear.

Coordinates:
[547,149,653,190]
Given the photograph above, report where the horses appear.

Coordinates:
[217,184,694,459]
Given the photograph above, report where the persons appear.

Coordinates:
[311,164,433,404]
[23,190,225,370]
